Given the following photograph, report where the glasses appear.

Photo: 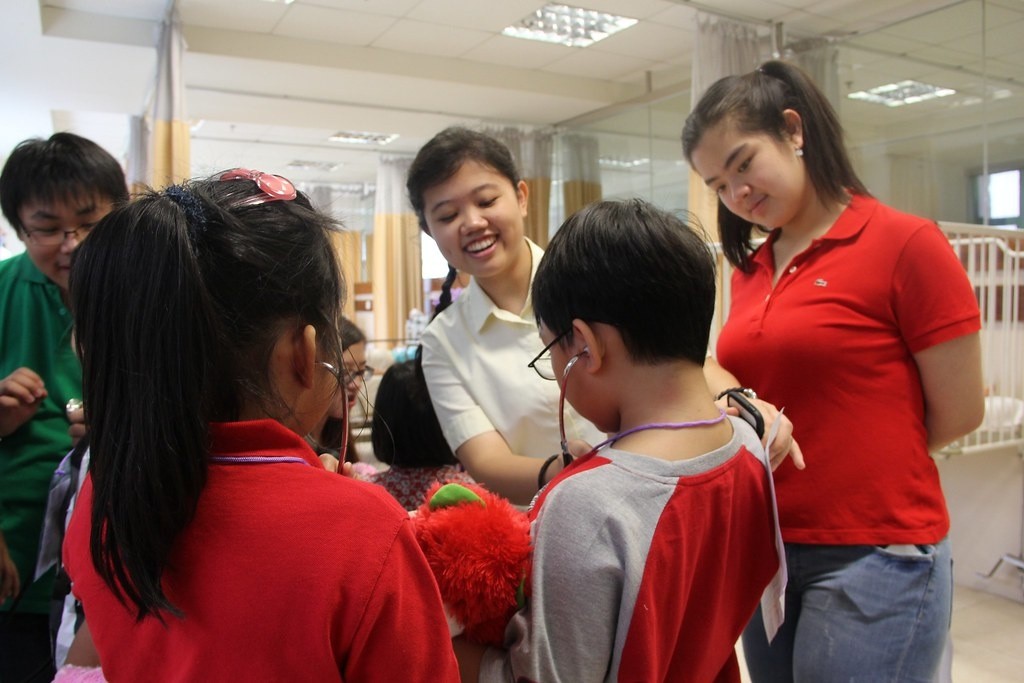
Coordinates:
[339,366,375,385]
[528,328,577,382]
[14,212,97,246]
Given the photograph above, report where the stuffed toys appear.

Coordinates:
[402,474,539,632]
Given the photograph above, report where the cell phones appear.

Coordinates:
[727,392,765,440]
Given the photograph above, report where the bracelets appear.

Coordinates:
[539,452,563,491]
[715,386,760,403]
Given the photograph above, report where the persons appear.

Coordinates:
[315,313,368,473]
[680,61,987,683]
[529,199,807,683]
[1,130,130,683]
[72,166,460,683]
[407,124,806,502]
